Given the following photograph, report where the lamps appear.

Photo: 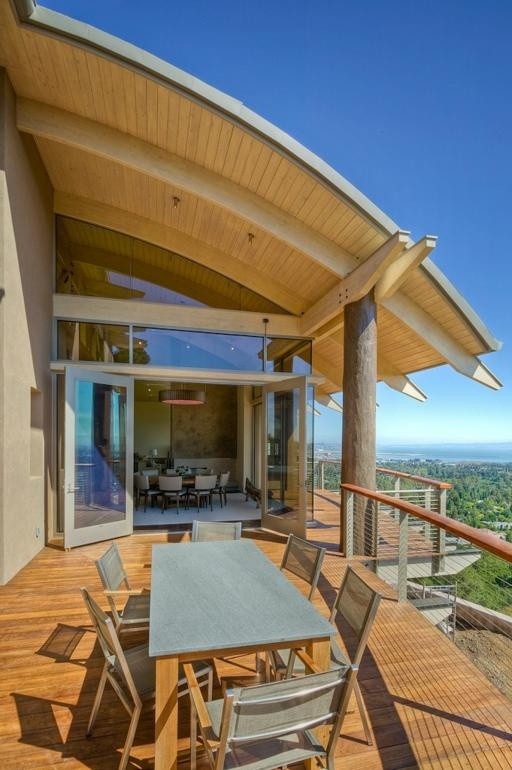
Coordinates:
[159,383,207,406]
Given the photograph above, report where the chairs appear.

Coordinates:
[134,465,231,515]
